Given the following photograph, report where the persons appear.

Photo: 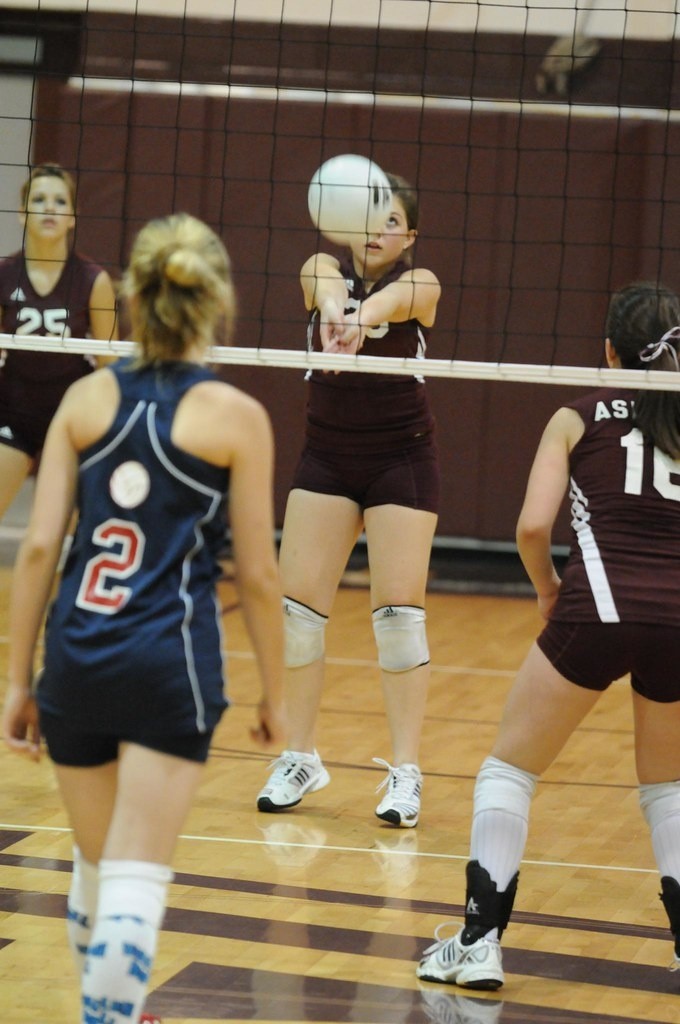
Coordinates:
[1,207,287,1024]
[0,162,120,603]
[253,173,444,829]
[415,283,680,991]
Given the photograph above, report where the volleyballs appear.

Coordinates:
[307,153,396,251]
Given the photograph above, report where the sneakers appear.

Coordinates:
[256,750,330,811]
[415,919,504,989]
[369,757,423,826]
